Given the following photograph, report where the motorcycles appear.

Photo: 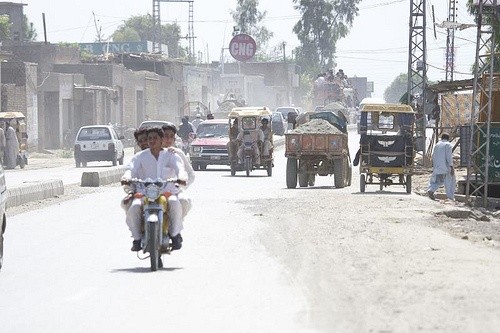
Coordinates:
[121,179,187,271]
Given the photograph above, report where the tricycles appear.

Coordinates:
[359,104,416,194]
[230,106,273,176]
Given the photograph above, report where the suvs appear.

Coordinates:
[189,119,238,170]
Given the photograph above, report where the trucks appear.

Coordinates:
[285,106,352,188]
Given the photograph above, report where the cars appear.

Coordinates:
[270,107,301,136]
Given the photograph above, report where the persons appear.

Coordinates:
[232,117,271,169]
[427,133,457,201]
[120,113,195,252]
[176,115,196,142]
[0,127,19,168]
[315,69,350,87]
[191,114,204,129]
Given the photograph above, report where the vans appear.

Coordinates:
[74,125,125,168]
[135,121,183,155]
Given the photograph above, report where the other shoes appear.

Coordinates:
[427,191,435,200]
[448,198,458,204]
[172,234,183,249]
[132,239,141,251]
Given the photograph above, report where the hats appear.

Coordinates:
[196,114,201,117]
